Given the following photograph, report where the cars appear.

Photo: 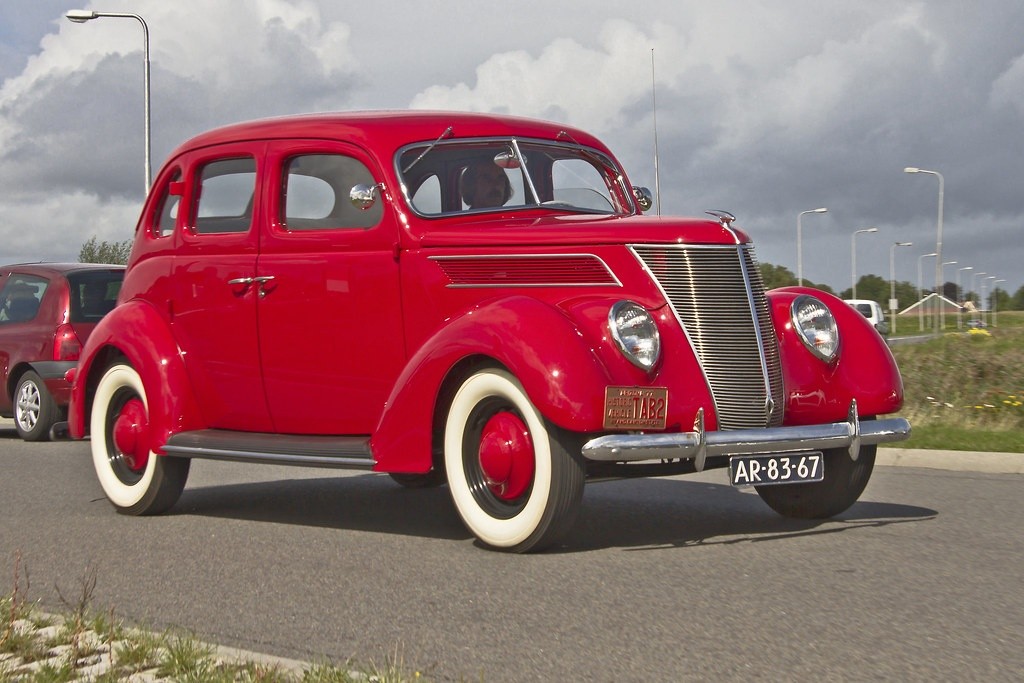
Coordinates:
[0,263,129,443]
[65,108,913,556]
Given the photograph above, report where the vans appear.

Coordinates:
[842,300,885,339]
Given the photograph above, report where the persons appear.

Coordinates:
[460,157,515,214]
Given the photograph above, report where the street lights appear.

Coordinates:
[917,253,1007,331]
[851,227,878,300]
[64,9,153,196]
[796,208,828,286]
[888,242,913,333]
[904,167,946,293]
